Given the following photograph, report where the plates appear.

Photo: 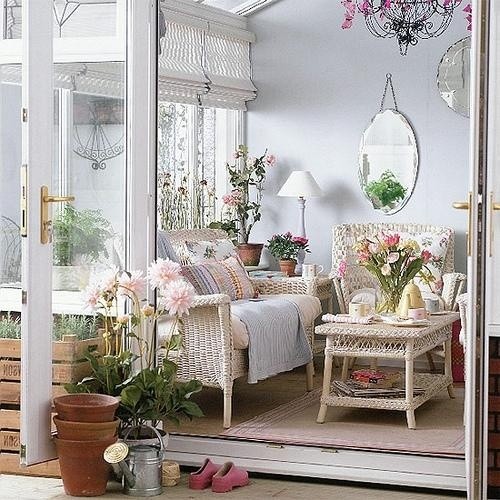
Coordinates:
[388,319,427,328]
[431,310,451,315]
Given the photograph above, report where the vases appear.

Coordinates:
[51,393,122,496]
[235,243,264,266]
[279,258,298,277]
[110,426,169,484]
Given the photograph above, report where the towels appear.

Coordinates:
[321,312,376,326]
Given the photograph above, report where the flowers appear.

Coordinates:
[264,231,311,260]
[62,256,207,439]
[208,145,277,244]
[351,226,433,313]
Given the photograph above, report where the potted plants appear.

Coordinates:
[52,203,116,291]
[365,169,407,209]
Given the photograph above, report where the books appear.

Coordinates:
[332,378,426,399]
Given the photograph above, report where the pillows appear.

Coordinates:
[379,225,452,310]
[180,255,258,301]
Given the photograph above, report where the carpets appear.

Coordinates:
[218,380,465,454]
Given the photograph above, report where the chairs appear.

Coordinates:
[328,222,468,374]
[157,228,324,429]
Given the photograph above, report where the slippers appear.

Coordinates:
[188,459,249,493]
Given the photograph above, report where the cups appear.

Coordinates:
[349,301,371,319]
[301,262,324,278]
[407,307,431,323]
[423,297,440,314]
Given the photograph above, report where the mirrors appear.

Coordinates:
[357,106,419,216]
[436,34,472,118]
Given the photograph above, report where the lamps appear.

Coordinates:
[338,0,463,56]
[276,170,325,275]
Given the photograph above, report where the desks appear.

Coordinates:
[248,272,341,368]
